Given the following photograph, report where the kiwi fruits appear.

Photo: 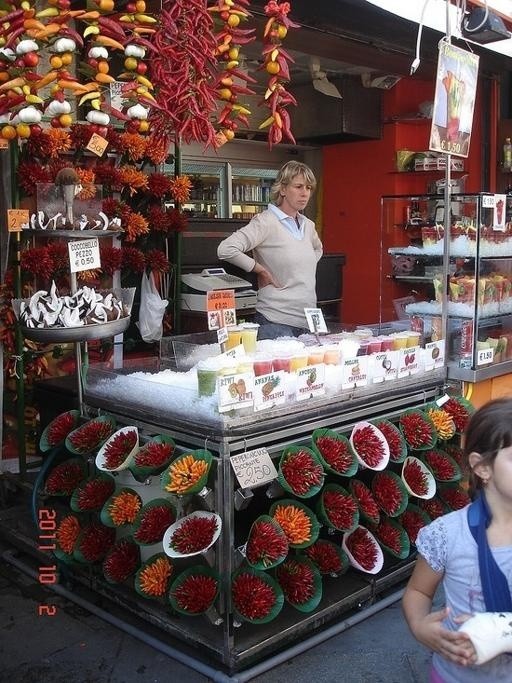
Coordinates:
[229,379,246,398]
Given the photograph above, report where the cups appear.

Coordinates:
[412,227,512,369]
[197,322,422,398]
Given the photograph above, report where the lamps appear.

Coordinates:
[307,60,401,101]
[460,8,512,45]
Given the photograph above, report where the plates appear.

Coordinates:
[21,229,121,239]
[22,315,130,343]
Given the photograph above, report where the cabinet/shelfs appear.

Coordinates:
[378,192,510,409]
[17,219,132,376]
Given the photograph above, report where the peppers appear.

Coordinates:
[46,413,216,612]
[233,399,469,620]
[0,0,297,376]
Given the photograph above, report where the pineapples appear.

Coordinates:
[262,377,280,395]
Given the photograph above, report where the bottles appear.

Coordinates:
[411,202,423,226]
[504,138,512,167]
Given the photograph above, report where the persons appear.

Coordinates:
[401,396,511,683]
[213,159,327,339]
